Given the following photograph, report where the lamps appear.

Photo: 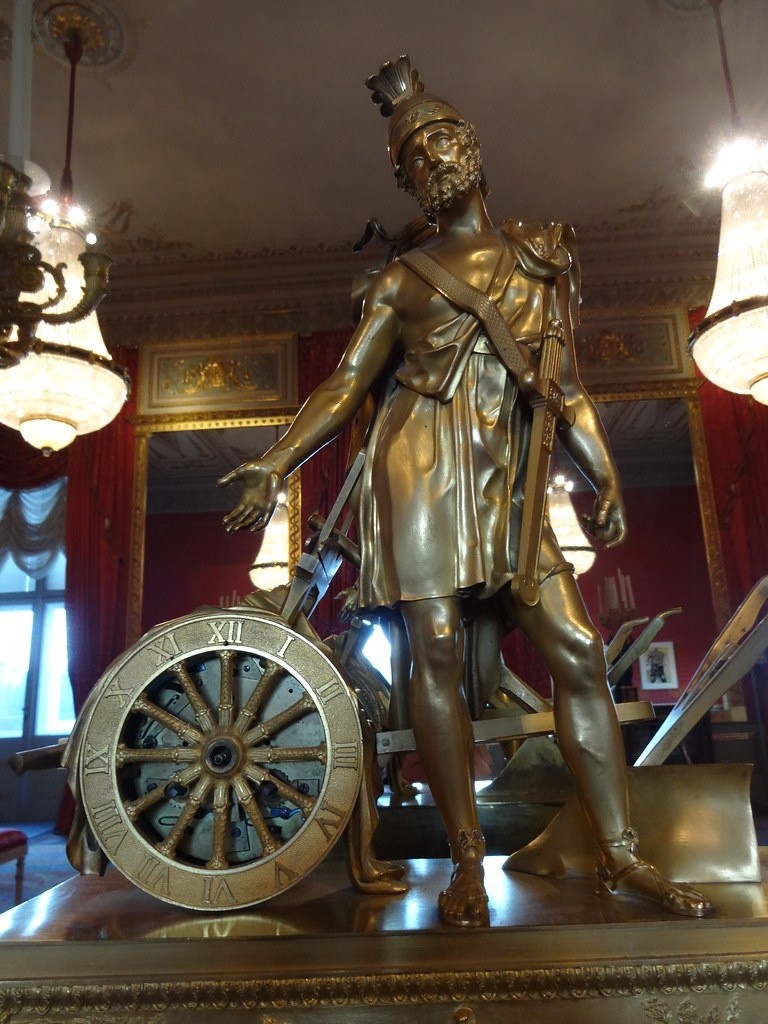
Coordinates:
[649,0,768,404]
[0,0,133,458]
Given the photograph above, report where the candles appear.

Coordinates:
[605,576,620,609]
[231,590,237,607]
[621,577,628,609]
[220,596,224,607]
[626,575,635,609]
[226,595,230,608]
[617,568,625,601]
[236,595,240,606]
[597,583,604,617]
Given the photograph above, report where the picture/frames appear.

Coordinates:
[639,641,679,690]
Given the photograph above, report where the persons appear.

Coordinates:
[219,52,720,932]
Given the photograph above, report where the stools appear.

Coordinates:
[0,829,29,905]
